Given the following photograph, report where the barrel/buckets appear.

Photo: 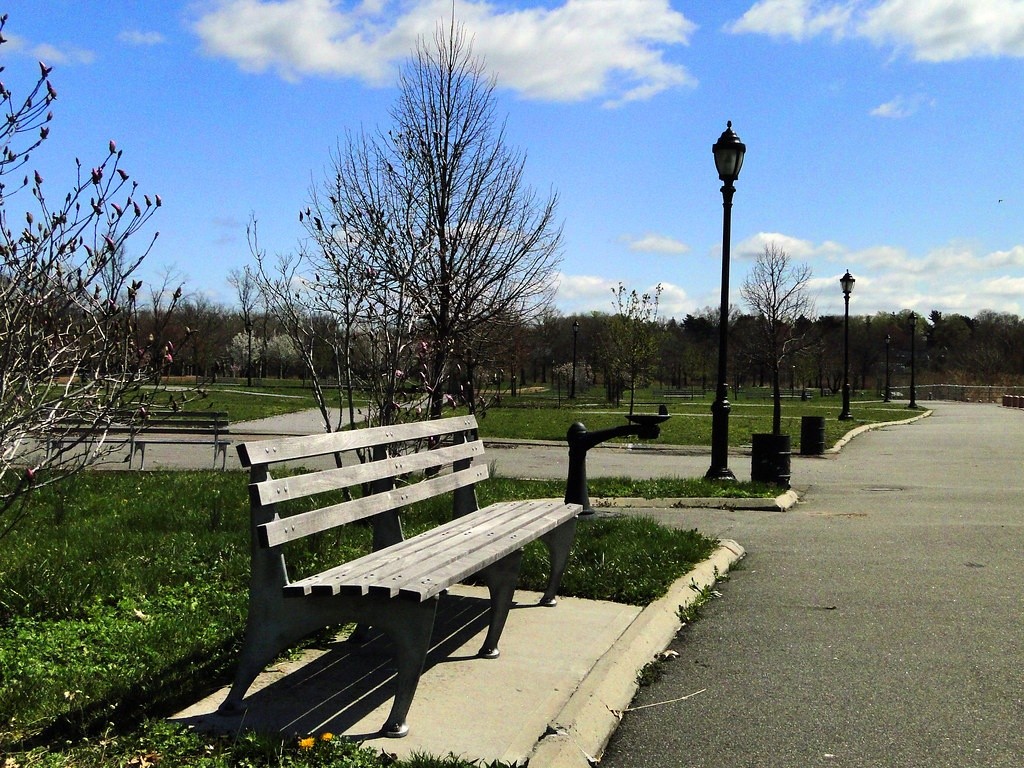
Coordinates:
[800,415,826,456]
[752,434,790,483]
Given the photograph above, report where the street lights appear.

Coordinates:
[907,310,919,408]
[701,120,747,485]
[570,320,580,399]
[884,334,891,402]
[839,269,857,421]
[246,318,253,386]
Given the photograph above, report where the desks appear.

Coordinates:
[624,414,672,439]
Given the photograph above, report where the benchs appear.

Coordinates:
[771,390,815,400]
[35,407,234,470]
[213,414,584,737]
[652,390,706,400]
[746,392,782,402]
[197,376,241,387]
